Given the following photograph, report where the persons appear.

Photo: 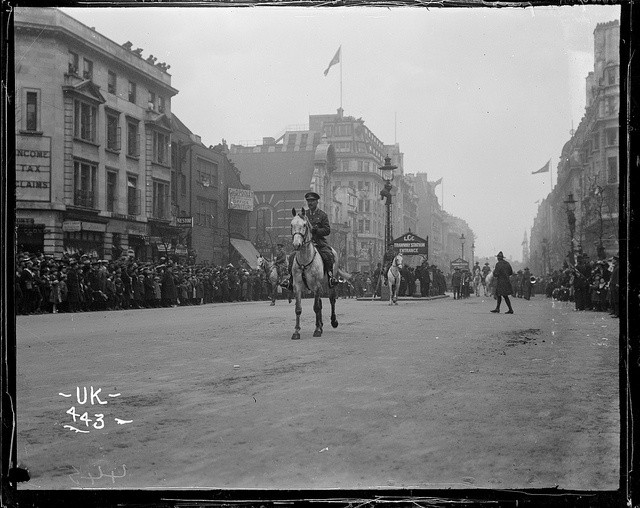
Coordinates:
[402,261,448,298]
[338,261,447,297]
[382,241,398,284]
[482,262,490,287]
[490,251,515,313]
[340,262,381,297]
[452,267,461,299]
[472,261,482,281]
[589,255,619,318]
[273,243,288,276]
[289,191,338,288]
[15,246,272,314]
[513,267,532,301]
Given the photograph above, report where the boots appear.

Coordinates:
[505,298,513,314]
[491,298,501,312]
[327,265,336,288]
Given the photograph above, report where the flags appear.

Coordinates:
[323,44,340,77]
[531,159,550,174]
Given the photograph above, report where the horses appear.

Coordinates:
[291,207,352,339]
[381,250,404,304]
[473,267,482,296]
[482,268,495,296]
[256,253,292,306]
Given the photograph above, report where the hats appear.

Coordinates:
[495,250,505,259]
[305,192,320,200]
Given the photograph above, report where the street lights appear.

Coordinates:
[470,243,476,267]
[378,153,398,251]
[458,234,466,260]
[342,222,350,272]
[563,191,578,269]
